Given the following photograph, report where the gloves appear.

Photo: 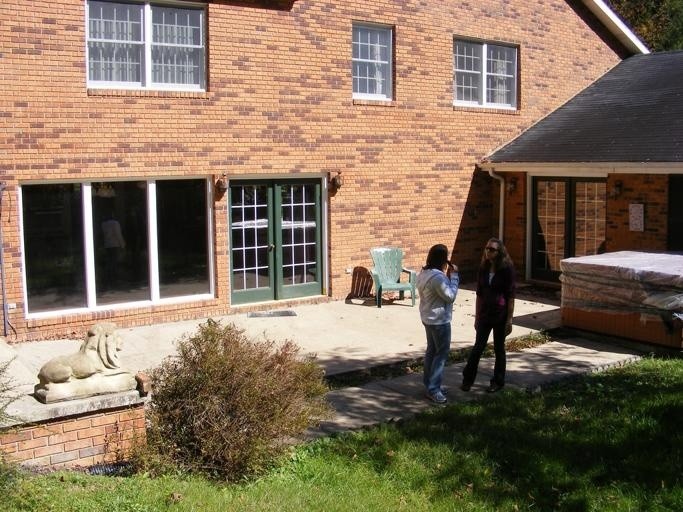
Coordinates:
[505,322,512,336]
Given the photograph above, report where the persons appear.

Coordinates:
[415,244,460,403]
[101,216,126,282]
[462,238,514,392]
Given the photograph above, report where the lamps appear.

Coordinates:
[96,182,117,199]
[333,168,345,190]
[217,172,229,193]
[613,178,623,200]
[506,176,520,196]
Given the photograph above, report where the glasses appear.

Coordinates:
[484,246,498,253]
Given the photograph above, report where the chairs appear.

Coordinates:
[370,247,417,308]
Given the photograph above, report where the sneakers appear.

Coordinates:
[462,381,471,391]
[488,382,501,393]
[426,385,448,403]
